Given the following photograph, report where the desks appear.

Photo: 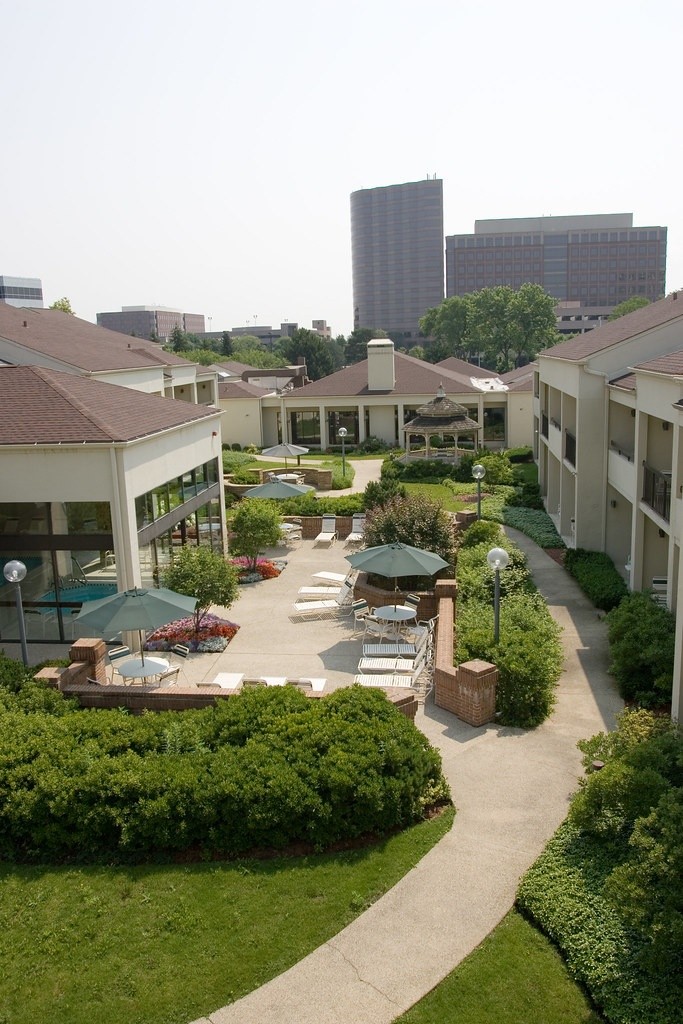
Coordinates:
[374,605,418,640]
[118,657,170,688]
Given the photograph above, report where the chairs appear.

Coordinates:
[353,650,435,702]
[655,470,672,505]
[108,646,135,686]
[266,470,306,485]
[277,518,303,550]
[240,677,288,688]
[140,549,152,572]
[310,565,353,588]
[344,513,366,550]
[154,644,190,688]
[352,599,378,635]
[24,607,59,640]
[314,513,339,550]
[291,577,355,622]
[363,626,428,659]
[297,569,358,603]
[196,673,248,690]
[357,649,435,676]
[650,576,668,607]
[558,504,560,517]
[361,612,396,644]
[570,517,576,536]
[104,550,116,572]
[625,554,631,572]
[85,677,116,688]
[287,678,328,692]
[403,618,435,644]
[393,594,421,634]
[70,608,96,641]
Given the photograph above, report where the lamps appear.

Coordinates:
[672,399,683,411]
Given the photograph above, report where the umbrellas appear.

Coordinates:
[343,541,450,640]
[261,441,309,477]
[73,587,199,688]
[240,477,316,500]
